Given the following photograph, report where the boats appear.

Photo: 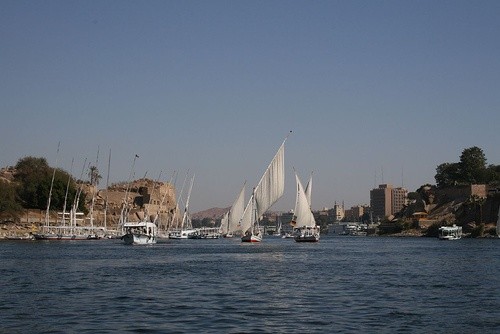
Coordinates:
[120,222,156,245]
[271,227,294,239]
[340,224,369,237]
[438,225,463,241]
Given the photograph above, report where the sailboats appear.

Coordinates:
[292,166,320,242]
[218,180,247,238]
[8,139,195,239]
[237,131,292,243]
